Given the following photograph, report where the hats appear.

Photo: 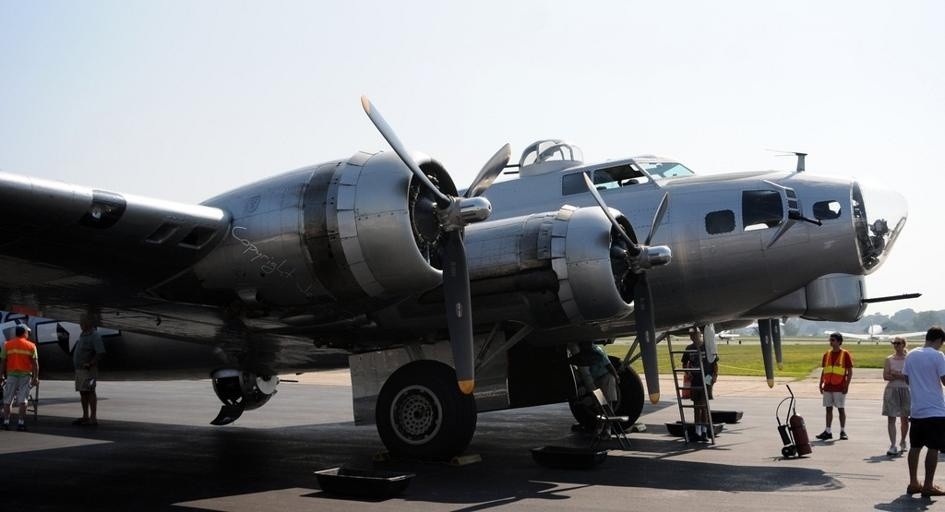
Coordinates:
[15,324,32,333]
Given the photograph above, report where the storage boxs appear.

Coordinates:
[710,410,743,424]
[665,422,723,438]
[528,446,606,470]
[314,468,416,499]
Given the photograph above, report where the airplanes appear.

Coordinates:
[824,324,929,347]
[0,92,910,466]
[716,330,744,345]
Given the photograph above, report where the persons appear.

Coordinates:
[900,327,945,499]
[681,327,718,442]
[883,337,911,455]
[590,344,621,440]
[73,319,106,426]
[816,332,854,440]
[0,324,40,431]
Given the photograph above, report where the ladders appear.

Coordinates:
[667,328,715,445]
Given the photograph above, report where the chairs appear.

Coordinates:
[591,388,632,450]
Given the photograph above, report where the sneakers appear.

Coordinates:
[91,418,98,426]
[0,424,8,430]
[840,431,847,439]
[921,486,945,497]
[71,418,89,422]
[694,433,702,441]
[887,447,897,455]
[907,482,923,494]
[701,431,707,441]
[816,431,832,439]
[16,424,25,431]
[900,441,907,452]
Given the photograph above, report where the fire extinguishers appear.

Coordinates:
[786,396,812,457]
